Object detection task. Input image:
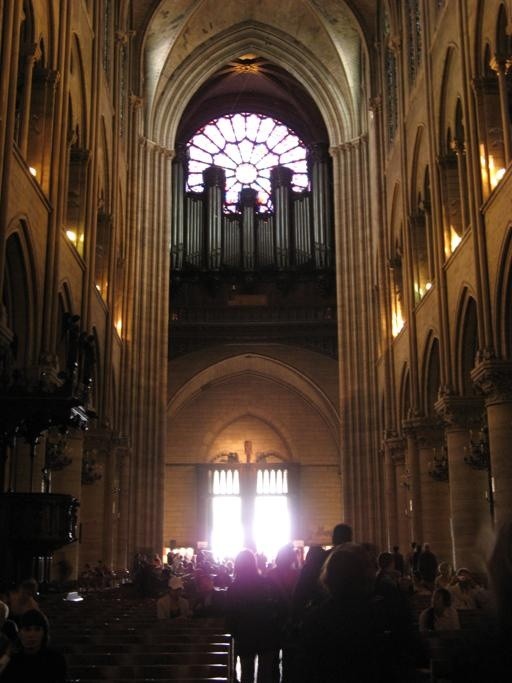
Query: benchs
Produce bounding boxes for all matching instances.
[0,583,239,683]
[406,590,481,683]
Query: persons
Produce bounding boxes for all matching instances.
[223,521,511,682]
[1,575,67,683]
[130,546,234,621]
[81,560,115,593]
